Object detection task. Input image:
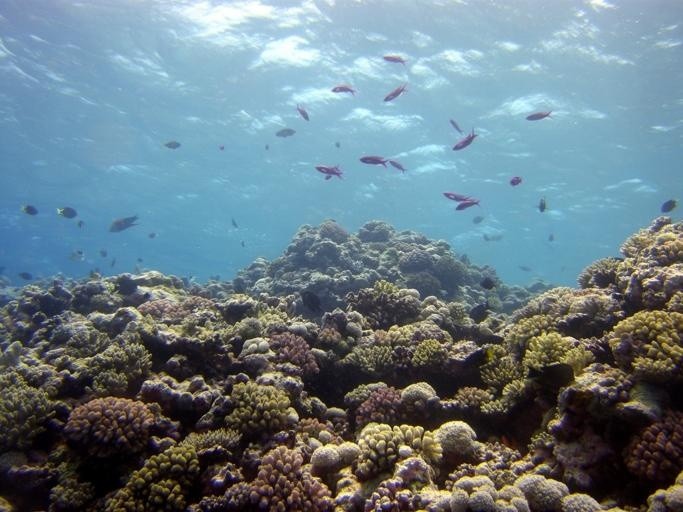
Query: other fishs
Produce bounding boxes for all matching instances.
[443,176,556,242]
